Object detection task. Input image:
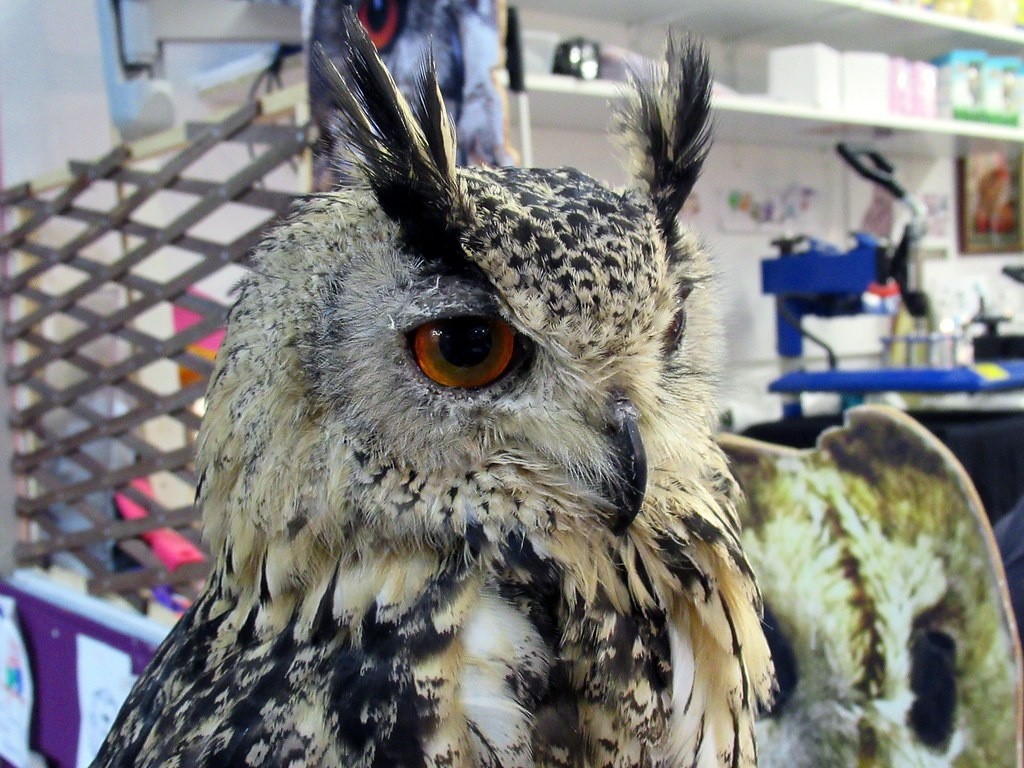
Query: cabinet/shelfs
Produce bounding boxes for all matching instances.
[509,1,1023,428]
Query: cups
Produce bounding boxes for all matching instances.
[553,38,600,74]
